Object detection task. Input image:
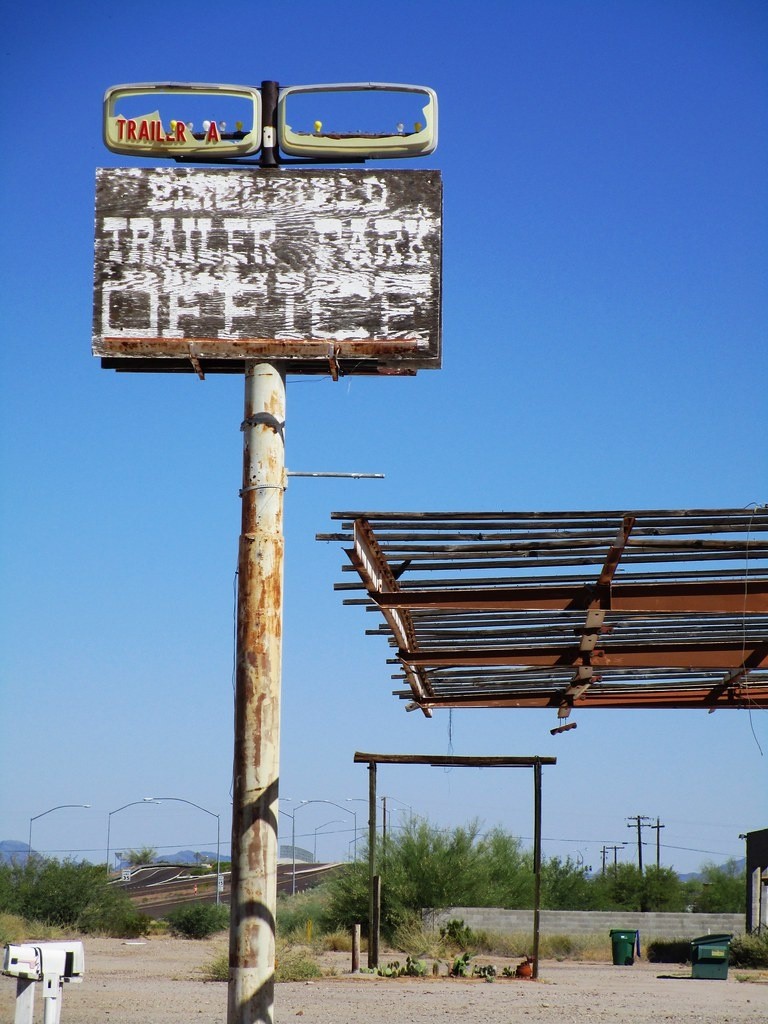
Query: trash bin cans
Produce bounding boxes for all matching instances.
[608,929,641,966]
[690,933,734,980]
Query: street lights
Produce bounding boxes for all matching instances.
[392,807,426,823]
[28,804,91,856]
[230,802,296,896]
[346,798,391,838]
[301,800,358,865]
[376,795,413,818]
[143,796,221,906]
[105,801,161,877]
